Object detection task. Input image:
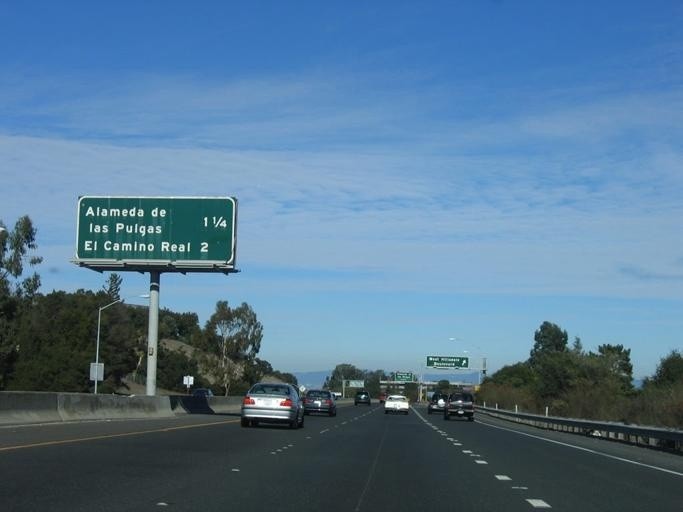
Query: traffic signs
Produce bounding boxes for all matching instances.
[427,356,469,369]
[75,196,235,266]
[395,372,413,382]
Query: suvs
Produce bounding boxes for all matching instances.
[428,393,448,414]
[444,393,475,421]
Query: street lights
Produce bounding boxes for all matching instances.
[95,295,149,393]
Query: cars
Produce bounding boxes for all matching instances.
[384,395,409,415]
[355,391,370,405]
[241,384,304,428]
[303,390,336,415]
[192,388,214,397]
